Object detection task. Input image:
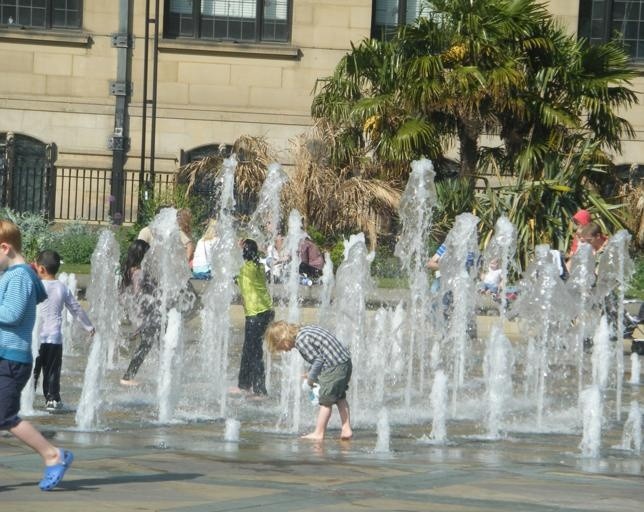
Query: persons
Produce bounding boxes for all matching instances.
[34,249,95,411]
[119,209,323,399]
[266,320,352,440]
[429,210,613,336]
[0,220,74,490]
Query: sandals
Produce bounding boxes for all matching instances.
[39,449,74,492]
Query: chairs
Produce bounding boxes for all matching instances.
[605,300,643,339]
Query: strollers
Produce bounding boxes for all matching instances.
[584,281,644,355]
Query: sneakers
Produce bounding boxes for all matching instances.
[45,400,63,411]
